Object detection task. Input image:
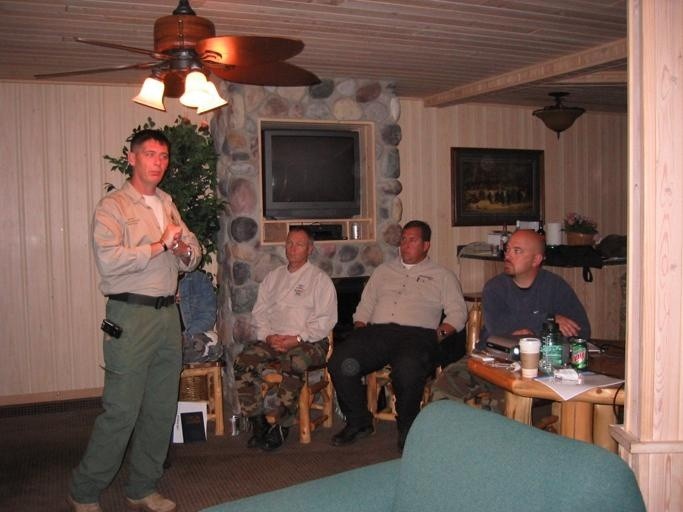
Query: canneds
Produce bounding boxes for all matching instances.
[568,337,589,373]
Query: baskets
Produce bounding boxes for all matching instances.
[179,367,219,426]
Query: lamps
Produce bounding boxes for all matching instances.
[131,67,166,111]
[180,61,210,107]
[196,80,228,115]
[532,92,586,140]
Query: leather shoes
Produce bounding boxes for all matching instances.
[122,491,176,512]
[64,491,104,512]
[330,417,376,447]
[397,432,410,452]
[246,414,270,448]
[263,423,288,452]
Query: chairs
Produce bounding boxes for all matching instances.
[466,305,489,409]
[261,329,334,444]
[365,364,442,421]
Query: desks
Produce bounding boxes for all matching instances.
[466,357,624,455]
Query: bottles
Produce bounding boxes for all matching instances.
[539,318,563,373]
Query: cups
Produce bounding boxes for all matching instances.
[518,338,540,378]
[544,223,563,246]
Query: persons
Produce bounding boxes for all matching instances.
[430,229,591,416]
[163,268,217,469]
[327,220,469,454]
[66,128,202,512]
[234,227,338,450]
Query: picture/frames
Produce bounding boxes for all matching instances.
[451,146,545,226]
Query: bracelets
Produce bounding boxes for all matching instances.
[184,246,192,257]
[160,240,168,251]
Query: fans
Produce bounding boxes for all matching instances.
[33,1,322,98]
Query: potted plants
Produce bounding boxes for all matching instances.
[104,115,235,401]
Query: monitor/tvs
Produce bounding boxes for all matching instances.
[261,129,361,220]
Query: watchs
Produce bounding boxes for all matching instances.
[438,328,448,339]
[297,335,303,345]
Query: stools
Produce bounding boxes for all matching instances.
[173,400,209,444]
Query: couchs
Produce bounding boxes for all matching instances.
[198,399,646,512]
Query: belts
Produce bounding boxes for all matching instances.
[106,290,176,310]
